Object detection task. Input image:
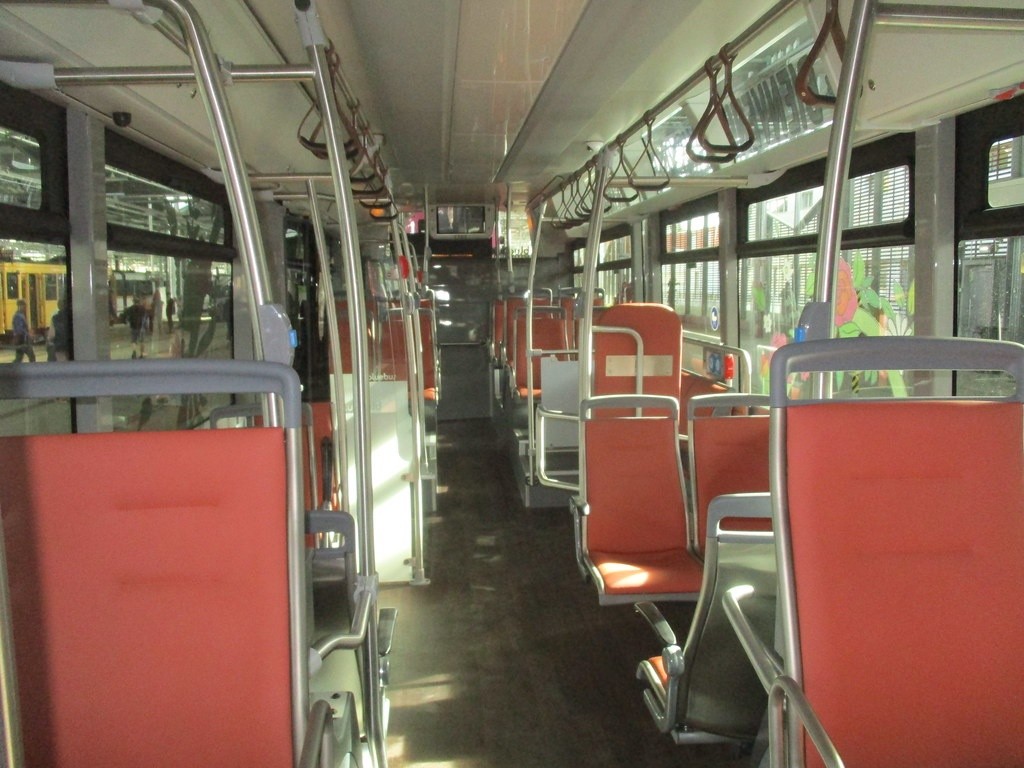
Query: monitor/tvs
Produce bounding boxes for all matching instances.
[428,203,495,240]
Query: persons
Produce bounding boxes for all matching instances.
[12,300,35,363]
[110,290,176,360]
[48,298,70,361]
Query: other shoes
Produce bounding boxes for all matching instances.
[132,351,136,359]
[139,355,144,359]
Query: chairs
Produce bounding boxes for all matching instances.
[489,287,1024,768]
[0,239,440,768]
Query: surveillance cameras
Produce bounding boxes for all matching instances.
[111,112,132,127]
[587,142,603,152]
